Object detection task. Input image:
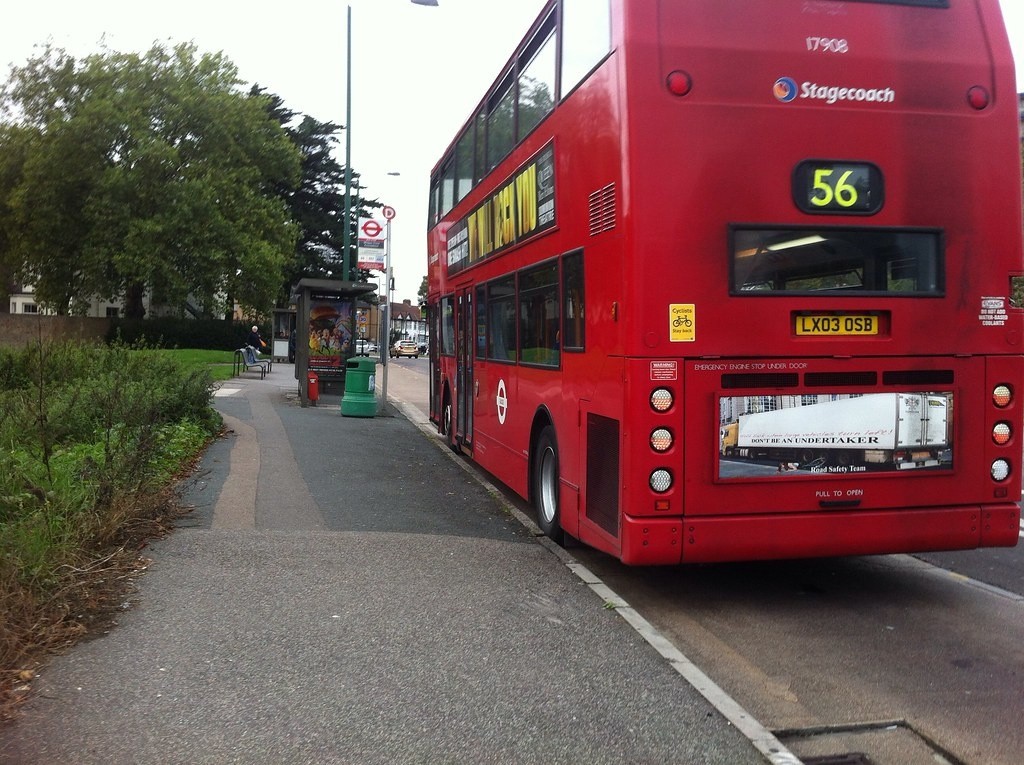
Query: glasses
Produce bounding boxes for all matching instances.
[344,342,349,345]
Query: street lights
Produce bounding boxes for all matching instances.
[343,0,440,282]
[355,172,401,283]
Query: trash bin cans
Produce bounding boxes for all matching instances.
[340,356,378,417]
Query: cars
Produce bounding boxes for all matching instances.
[356,339,369,357]
[369,343,380,352]
[389,340,419,359]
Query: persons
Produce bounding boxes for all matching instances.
[247,326,262,363]
[310,327,342,356]
[285,331,297,364]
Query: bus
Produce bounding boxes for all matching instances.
[420,0,1024,568]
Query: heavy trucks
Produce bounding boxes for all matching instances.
[722,391,948,471]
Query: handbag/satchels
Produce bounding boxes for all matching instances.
[260,339,266,347]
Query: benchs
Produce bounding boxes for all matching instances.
[233,348,266,380]
[246,345,272,373]
